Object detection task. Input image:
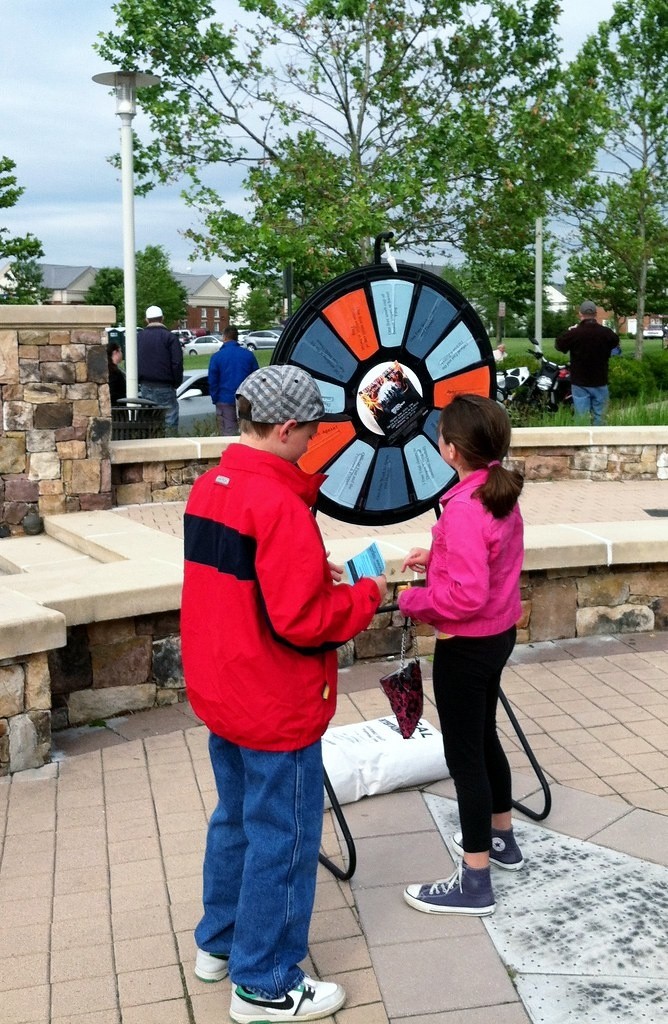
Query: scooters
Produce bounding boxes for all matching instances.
[497,366,531,409]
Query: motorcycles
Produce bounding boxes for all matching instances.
[523,334,576,412]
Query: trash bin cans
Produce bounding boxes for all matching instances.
[111,398,169,440]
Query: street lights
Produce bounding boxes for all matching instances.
[91,66,167,421]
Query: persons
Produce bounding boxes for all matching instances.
[208,325,259,436]
[107,340,126,406]
[137,306,183,438]
[359,372,408,415]
[555,301,621,424]
[493,344,507,362]
[178,364,387,1024]
[397,392,526,917]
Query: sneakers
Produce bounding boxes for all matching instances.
[403,859,496,917]
[452,826,524,871]
[229,969,347,1024]
[194,949,231,983]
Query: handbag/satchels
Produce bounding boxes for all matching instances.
[380,615,424,739]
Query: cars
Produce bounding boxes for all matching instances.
[183,334,248,356]
[170,327,250,346]
[172,369,238,418]
[642,324,665,339]
[242,329,284,352]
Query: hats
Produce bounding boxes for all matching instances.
[146,305,162,319]
[234,365,353,424]
[580,301,596,314]
